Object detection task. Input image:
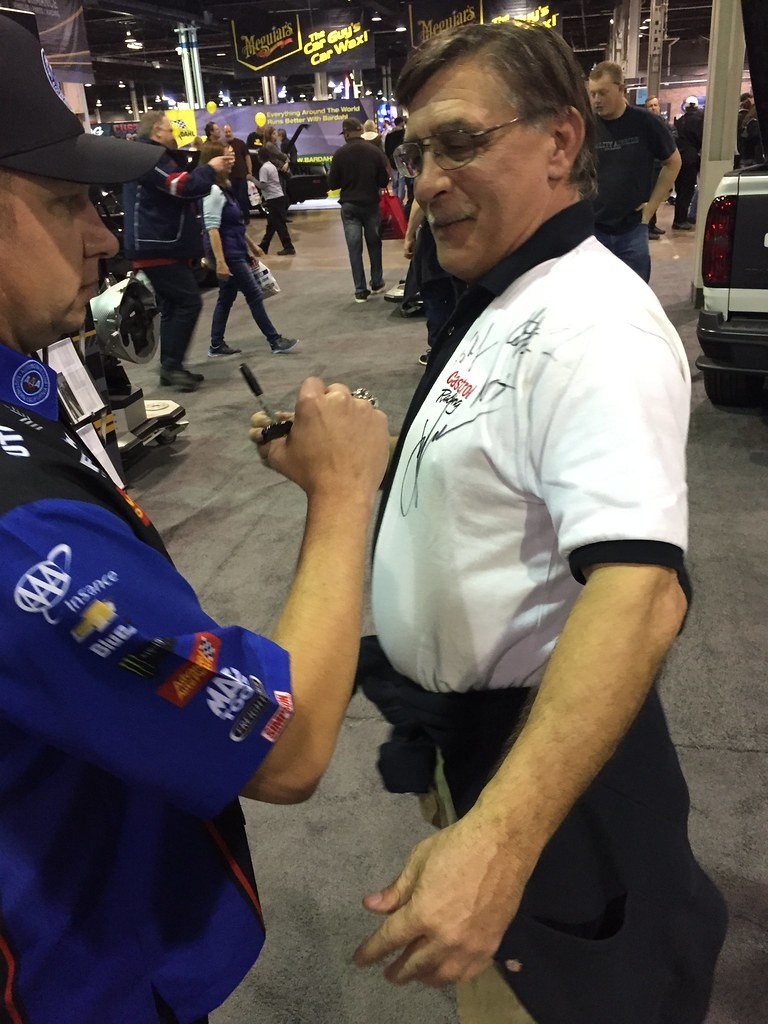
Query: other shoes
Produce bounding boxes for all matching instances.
[672,221,692,231]
[159,365,204,389]
[648,225,665,240]
[276,248,296,256]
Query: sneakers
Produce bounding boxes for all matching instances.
[419,348,432,365]
[270,335,301,354]
[355,289,371,304]
[208,341,242,359]
[371,281,386,294]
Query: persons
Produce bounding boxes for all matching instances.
[587,61,682,283]
[359,115,414,210]
[190,121,311,358]
[249,22,730,1024]
[403,197,468,365]
[124,111,237,392]
[643,92,764,241]
[1,6,388,1024]
[327,118,391,303]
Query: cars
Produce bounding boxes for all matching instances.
[247,121,332,218]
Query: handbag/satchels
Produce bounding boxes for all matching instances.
[376,186,408,241]
[248,255,282,301]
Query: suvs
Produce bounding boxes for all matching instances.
[690,166,767,411]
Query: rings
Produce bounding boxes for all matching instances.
[350,388,379,409]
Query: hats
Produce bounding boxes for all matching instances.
[1,11,169,185]
[684,95,698,108]
[338,117,363,137]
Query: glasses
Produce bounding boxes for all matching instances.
[389,107,560,179]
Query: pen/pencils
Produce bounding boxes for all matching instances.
[260,419,292,441]
[238,362,274,420]
